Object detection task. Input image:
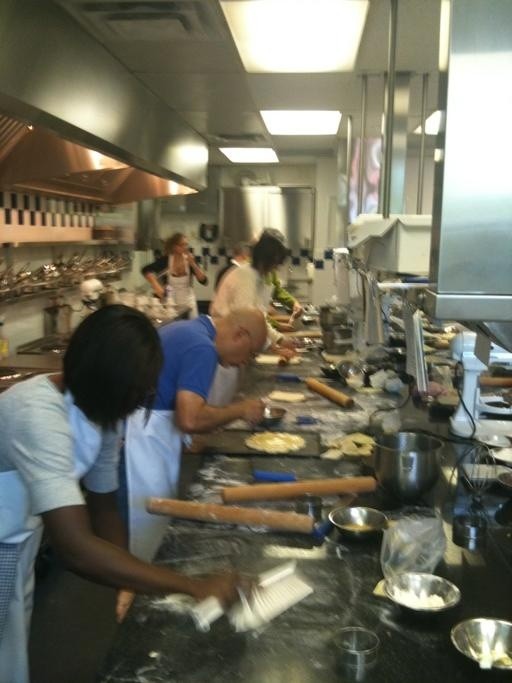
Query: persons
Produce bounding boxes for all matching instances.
[201,226,302,469]
[142,232,209,320]
[80,304,268,637]
[0,303,259,683]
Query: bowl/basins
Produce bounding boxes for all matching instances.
[337,358,365,380]
[256,405,286,426]
[318,363,338,379]
[383,571,464,616]
[323,326,353,353]
[446,616,512,675]
[320,306,346,330]
[327,503,386,539]
[374,431,446,498]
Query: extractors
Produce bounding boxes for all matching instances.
[2,2,209,206]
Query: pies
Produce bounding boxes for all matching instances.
[245,431,307,453]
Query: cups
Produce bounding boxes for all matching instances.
[329,626,381,682]
[295,493,322,521]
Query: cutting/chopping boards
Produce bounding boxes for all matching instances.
[278,330,322,339]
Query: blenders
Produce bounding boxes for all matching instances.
[449,328,511,446]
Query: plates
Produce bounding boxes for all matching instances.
[383,312,461,411]
[453,536,489,550]
[463,390,512,486]
[453,515,488,537]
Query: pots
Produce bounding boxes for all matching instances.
[2,248,139,298]
